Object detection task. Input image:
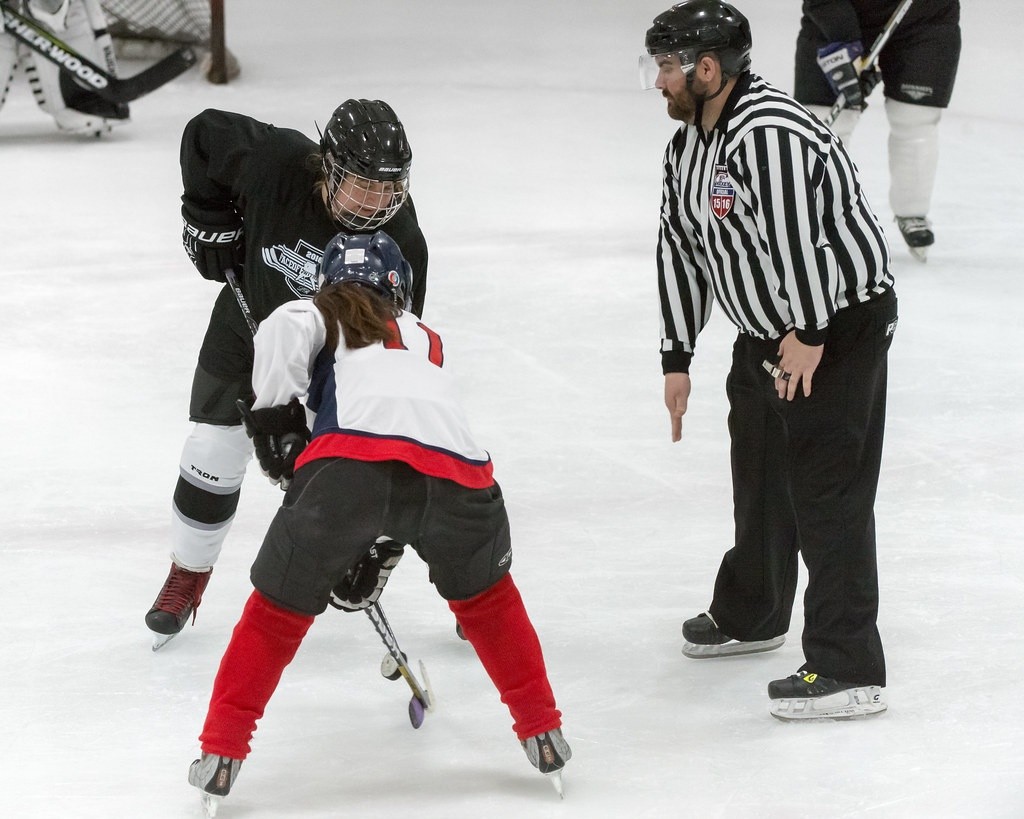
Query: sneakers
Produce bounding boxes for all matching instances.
[145,561,213,652]
[521,726,572,799]
[893,215,935,263]
[682,611,787,659]
[188,751,243,819]
[768,664,887,718]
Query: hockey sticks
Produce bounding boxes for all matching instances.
[0,1,199,107]
[58,0,131,119]
[823,1,914,128]
[225,264,425,732]
[235,398,441,714]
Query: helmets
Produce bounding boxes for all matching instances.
[319,230,413,309]
[645,0,752,78]
[314,99,416,230]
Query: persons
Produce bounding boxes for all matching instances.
[187,229,573,817]
[145,98,428,652]
[638,0,899,721]
[793,0,961,261]
[0,1,122,138]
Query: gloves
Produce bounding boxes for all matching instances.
[181,194,246,283]
[816,40,882,104]
[329,539,404,612]
[236,397,312,491]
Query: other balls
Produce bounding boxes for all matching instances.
[381,650,408,681]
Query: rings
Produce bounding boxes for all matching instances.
[762,359,792,381]
